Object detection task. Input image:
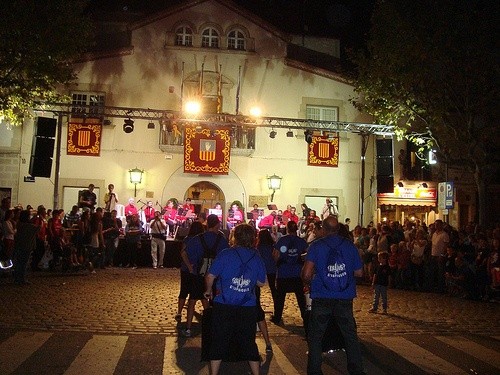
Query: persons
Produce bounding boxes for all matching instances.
[0,198,500,375]
[80,183,96,211]
[104,184,118,212]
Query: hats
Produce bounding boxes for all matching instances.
[207,214,222,224]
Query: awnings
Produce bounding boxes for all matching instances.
[377,197,436,207]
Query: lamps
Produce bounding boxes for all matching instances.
[327,130,334,140]
[422,182,428,189]
[286,128,293,137]
[303,129,313,145]
[147,119,155,130]
[397,181,404,188]
[128,165,145,197]
[122,117,134,134]
[266,172,283,202]
[164,154,173,160]
[268,127,277,138]
[210,125,218,137]
[195,122,202,129]
[227,125,235,136]
[103,117,112,126]
[167,121,174,134]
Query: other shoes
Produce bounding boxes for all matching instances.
[183,328,191,336]
[89,271,96,274]
[176,315,181,320]
[368,308,377,313]
[266,346,272,352]
[88,262,93,269]
[153,266,157,268]
[270,315,281,322]
[160,265,163,268]
[381,308,386,313]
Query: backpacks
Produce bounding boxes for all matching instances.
[199,233,222,281]
[318,237,351,292]
[221,246,258,305]
[282,237,302,264]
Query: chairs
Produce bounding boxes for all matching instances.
[138,210,170,238]
[115,204,127,226]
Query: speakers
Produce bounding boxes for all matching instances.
[375,138,394,194]
[28,116,57,178]
